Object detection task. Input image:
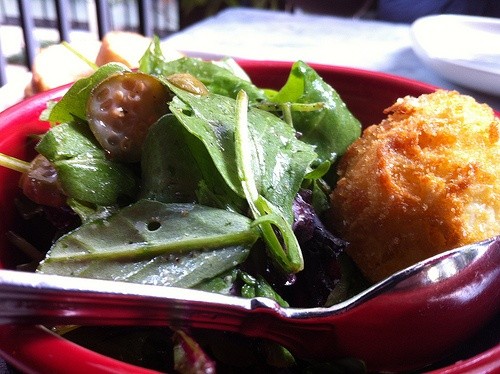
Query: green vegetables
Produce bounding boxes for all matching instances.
[0,30,364,374]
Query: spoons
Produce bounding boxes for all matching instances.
[0,234,499,373]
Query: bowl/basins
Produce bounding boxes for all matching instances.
[1,57,500,373]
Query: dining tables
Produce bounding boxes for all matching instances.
[0,8,500,373]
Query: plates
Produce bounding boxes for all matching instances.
[411,13,500,96]
